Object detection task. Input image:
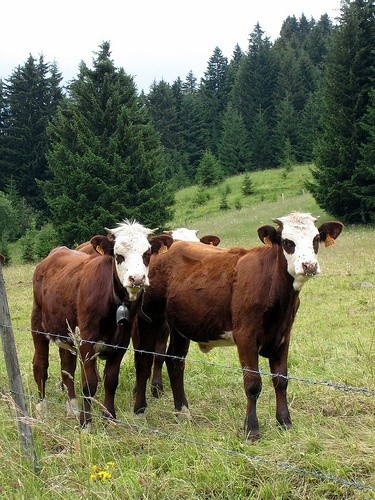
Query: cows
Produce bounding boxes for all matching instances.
[30,211,344,445]
